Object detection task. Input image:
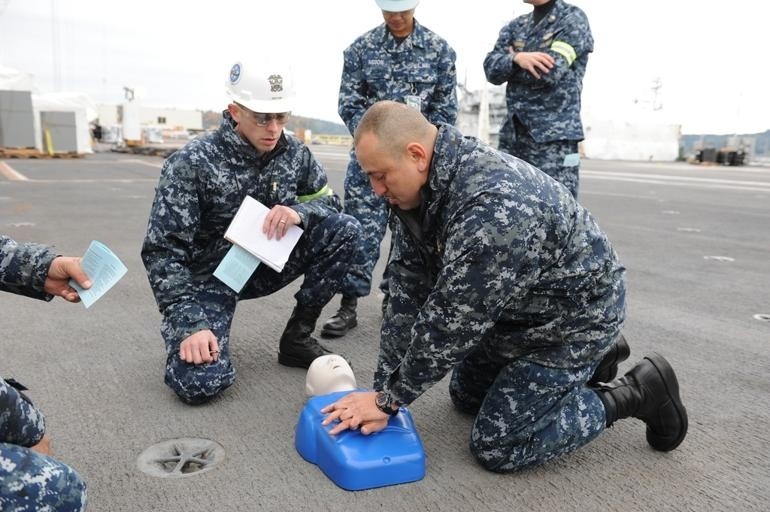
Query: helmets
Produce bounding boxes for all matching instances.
[374,0,420,13]
[226,58,300,115]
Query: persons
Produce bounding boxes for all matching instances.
[319,101,688,476]
[0,230,92,512]
[295,353,426,490]
[138,59,363,407]
[321,0,460,339]
[481,0,595,201]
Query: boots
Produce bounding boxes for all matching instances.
[320,295,360,339]
[593,334,631,384]
[590,352,690,452]
[277,305,355,377]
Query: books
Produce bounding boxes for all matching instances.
[68,238,128,309]
[212,243,262,294]
[222,193,305,274]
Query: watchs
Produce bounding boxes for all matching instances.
[374,390,399,417]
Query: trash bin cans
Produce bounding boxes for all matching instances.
[696,147,746,166]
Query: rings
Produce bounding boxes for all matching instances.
[280,218,287,224]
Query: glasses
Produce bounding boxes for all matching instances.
[230,102,292,127]
